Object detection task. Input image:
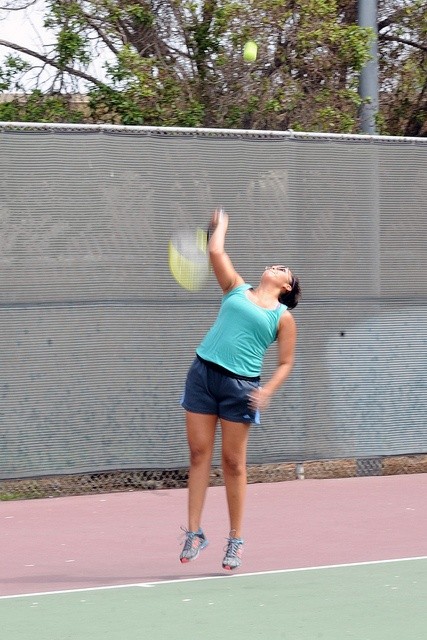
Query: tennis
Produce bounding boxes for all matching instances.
[243,42,257,62]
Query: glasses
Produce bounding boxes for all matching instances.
[265,266,290,284]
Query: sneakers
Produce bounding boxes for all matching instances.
[222,530,243,569]
[179,526,208,563]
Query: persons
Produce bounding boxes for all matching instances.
[180,208,299,570]
